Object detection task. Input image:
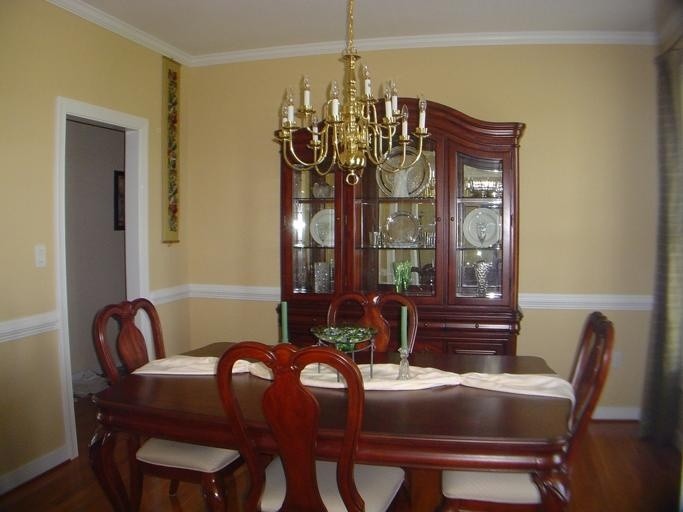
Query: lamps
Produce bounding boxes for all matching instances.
[274,1,433,187]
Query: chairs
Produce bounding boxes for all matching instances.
[90,286,615,511]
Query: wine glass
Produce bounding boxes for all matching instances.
[401,259,412,289]
[392,261,404,290]
[476,223,487,248]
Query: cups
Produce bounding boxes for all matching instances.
[368,231,390,248]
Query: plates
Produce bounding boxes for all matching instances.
[376,145,432,197]
[309,208,335,247]
[462,207,502,248]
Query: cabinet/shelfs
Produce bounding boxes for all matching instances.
[276,96,527,356]
